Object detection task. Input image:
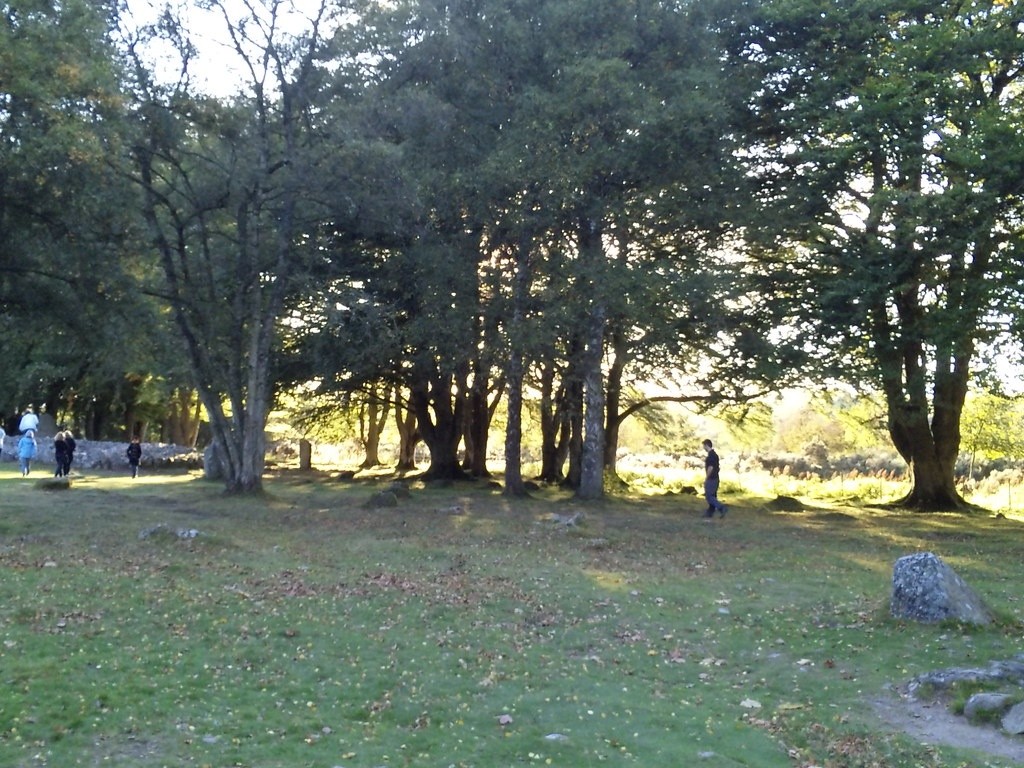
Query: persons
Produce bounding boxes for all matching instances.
[701,439,727,518]
[54,431,69,477]
[19,411,39,435]
[127,436,142,479]
[64,430,75,476]
[17,430,38,476]
